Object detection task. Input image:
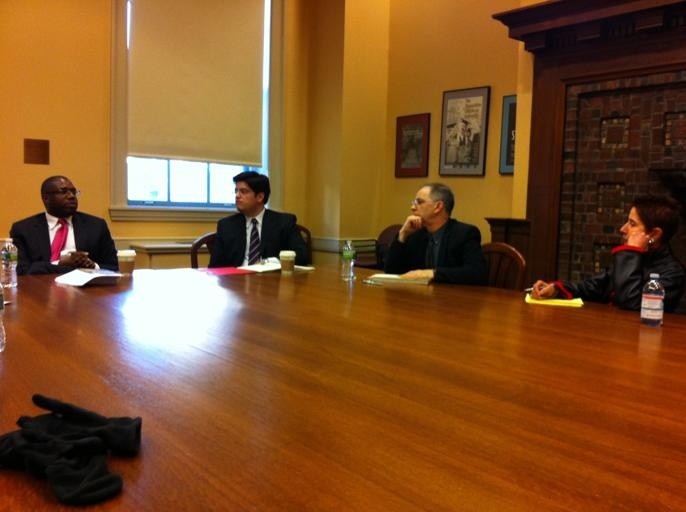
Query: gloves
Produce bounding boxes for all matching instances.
[0,416,123,507]
[18,393,141,459]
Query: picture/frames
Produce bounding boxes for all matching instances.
[394,112,431,178]
[498,94,517,175]
[438,85,491,177]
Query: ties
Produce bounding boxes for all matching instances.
[248,217,260,266]
[50,219,69,262]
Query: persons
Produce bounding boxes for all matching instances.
[10,176,119,274]
[459,119,467,163]
[209,171,312,267]
[385,182,488,285]
[465,128,474,149]
[446,123,458,163]
[532,198,679,313]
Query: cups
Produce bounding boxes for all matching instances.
[117,249,137,274]
[279,250,297,276]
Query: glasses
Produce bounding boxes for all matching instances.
[43,189,81,198]
[410,199,435,206]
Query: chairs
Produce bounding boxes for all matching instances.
[189,231,217,268]
[481,240,527,289]
[295,223,313,266]
[354,222,403,269]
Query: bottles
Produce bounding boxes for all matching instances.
[341,240,357,281]
[0,284,7,353]
[640,273,665,327]
[0,238,18,289]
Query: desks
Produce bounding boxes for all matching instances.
[129,240,209,270]
[0,263,686,511]
